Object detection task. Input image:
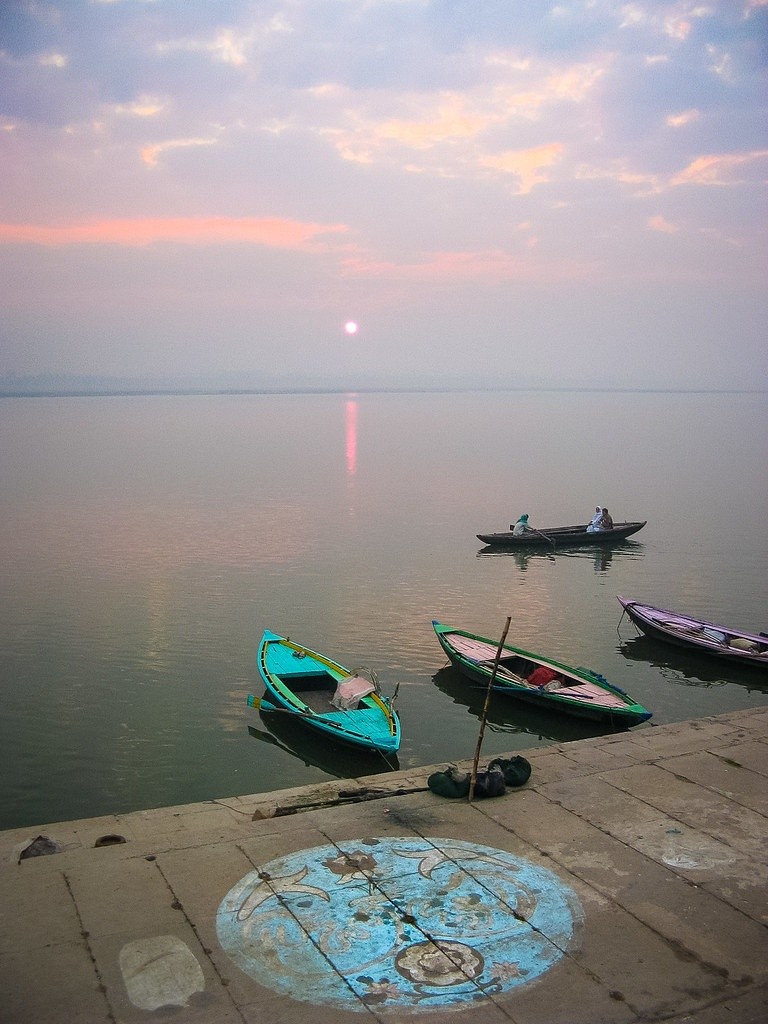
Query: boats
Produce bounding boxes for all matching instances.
[432,620,653,719]
[476,521,647,544]
[616,595,768,670]
[257,630,401,753]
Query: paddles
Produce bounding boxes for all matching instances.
[473,685,594,700]
[707,649,767,658]
[245,694,343,726]
[529,526,554,542]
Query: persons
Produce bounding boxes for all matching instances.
[586,505,614,532]
[512,514,536,536]
